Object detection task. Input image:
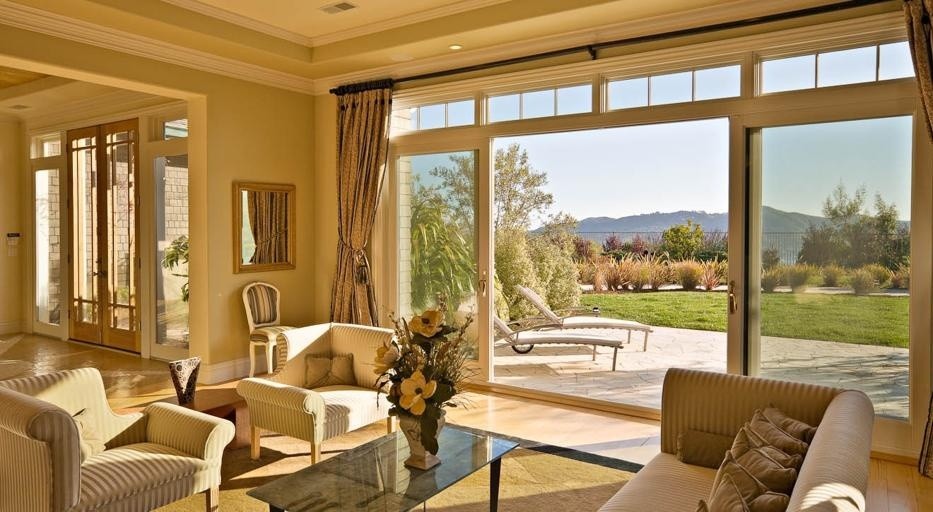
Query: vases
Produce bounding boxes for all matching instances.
[399,410,448,470]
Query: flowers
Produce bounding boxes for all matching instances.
[371,294,477,458]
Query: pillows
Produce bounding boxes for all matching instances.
[673,397,820,512]
[303,353,354,389]
[70,407,105,464]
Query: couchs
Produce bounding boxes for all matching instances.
[596,365,876,511]
[229,321,397,465]
[1,365,236,511]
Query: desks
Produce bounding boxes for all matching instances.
[111,387,249,429]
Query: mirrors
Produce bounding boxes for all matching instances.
[230,180,296,272]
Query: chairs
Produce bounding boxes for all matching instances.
[493,313,625,370]
[513,284,655,350]
[242,281,295,377]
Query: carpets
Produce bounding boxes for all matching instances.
[147,410,635,512]
